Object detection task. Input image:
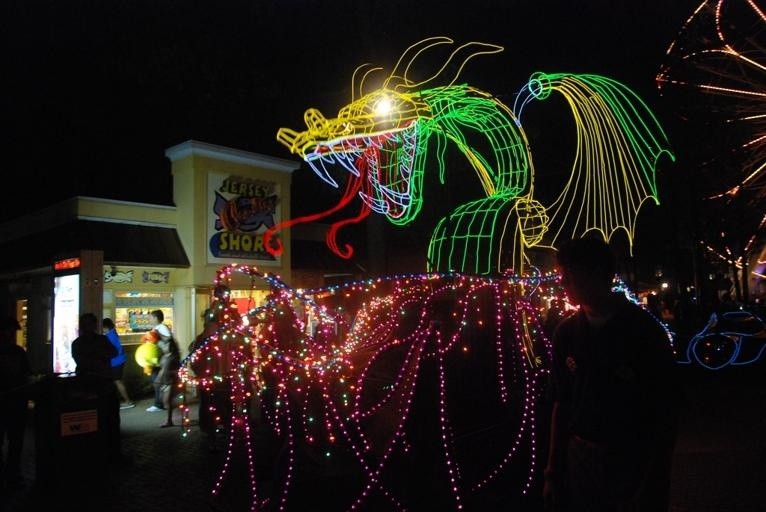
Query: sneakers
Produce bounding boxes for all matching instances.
[119,403,135,409]
[159,419,173,427]
[145,406,163,412]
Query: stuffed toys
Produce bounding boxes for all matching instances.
[134,330,160,376]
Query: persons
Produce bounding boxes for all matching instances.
[181,305,355,444]
[0,311,33,489]
[153,341,190,429]
[639,276,766,334]
[546,236,692,512]
[102,317,136,410]
[144,309,177,412]
[71,312,134,466]
